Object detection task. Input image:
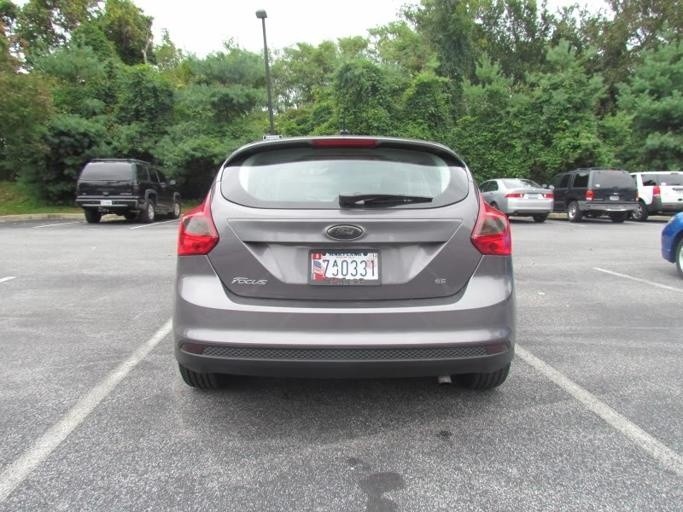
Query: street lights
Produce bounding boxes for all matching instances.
[255,10,277,136]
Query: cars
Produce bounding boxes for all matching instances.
[661,212,683,273]
[173,135,514,388]
[630,171,683,220]
[477,177,555,222]
[75,159,181,224]
[545,166,639,222]
